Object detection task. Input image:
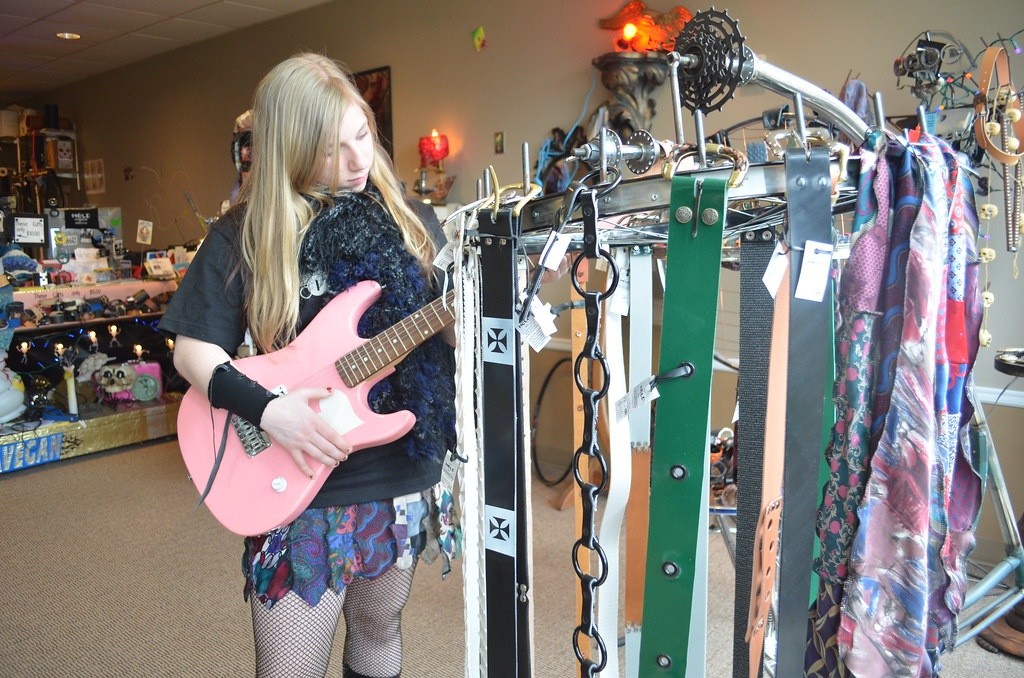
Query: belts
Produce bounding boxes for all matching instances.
[974,47,1024,166]
[438,113,831,678]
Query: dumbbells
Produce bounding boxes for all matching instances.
[570,128,665,175]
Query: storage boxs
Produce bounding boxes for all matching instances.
[93,361,163,402]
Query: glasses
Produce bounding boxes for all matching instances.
[711,454,732,477]
[711,444,734,461]
[711,481,738,508]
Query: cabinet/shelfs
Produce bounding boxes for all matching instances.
[0,279,189,472]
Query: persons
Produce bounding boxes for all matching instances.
[156,52,569,678]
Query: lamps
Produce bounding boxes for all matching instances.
[592,0,694,139]
[413,129,456,206]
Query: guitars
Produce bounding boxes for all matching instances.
[175,250,578,539]
